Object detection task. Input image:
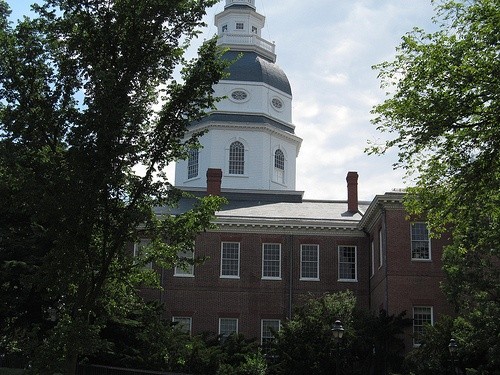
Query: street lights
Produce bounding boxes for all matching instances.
[449,339,457,375]
[332,320,345,375]
